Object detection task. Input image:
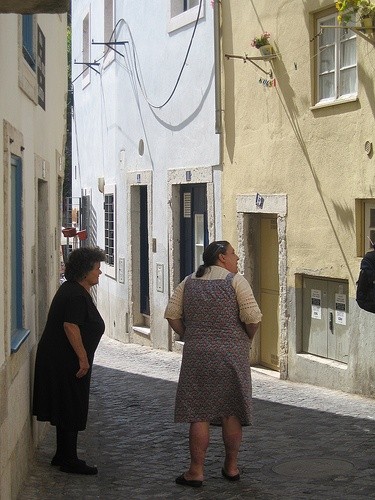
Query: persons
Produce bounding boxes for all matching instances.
[165,241,263,487]
[356,251,375,314]
[32,247,105,475]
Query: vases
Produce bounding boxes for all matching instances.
[258,45,273,55]
[360,18,373,26]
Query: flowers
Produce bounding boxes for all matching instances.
[335,0,375,25]
[250,32,271,49]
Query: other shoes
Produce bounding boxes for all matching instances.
[61,459,98,474]
[51,455,86,466]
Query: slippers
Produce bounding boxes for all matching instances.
[176,474,203,487]
[221,467,239,480]
